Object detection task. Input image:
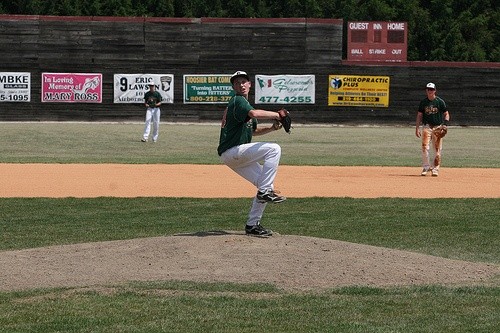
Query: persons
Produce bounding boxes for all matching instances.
[217,71,292,237]
[416,83,450,176]
[141,83,163,143]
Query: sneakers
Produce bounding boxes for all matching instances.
[256,188,286,204]
[245,220,273,238]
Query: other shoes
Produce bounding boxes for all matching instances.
[152,138,156,143]
[141,139,148,142]
[421,167,438,176]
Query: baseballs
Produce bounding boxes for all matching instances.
[273,122,281,130]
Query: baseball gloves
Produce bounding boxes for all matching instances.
[277,109,292,135]
[432,124,448,139]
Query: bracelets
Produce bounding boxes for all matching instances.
[442,120,449,126]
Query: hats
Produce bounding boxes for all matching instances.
[230,71,248,82]
[426,83,436,89]
[148,82,154,85]
